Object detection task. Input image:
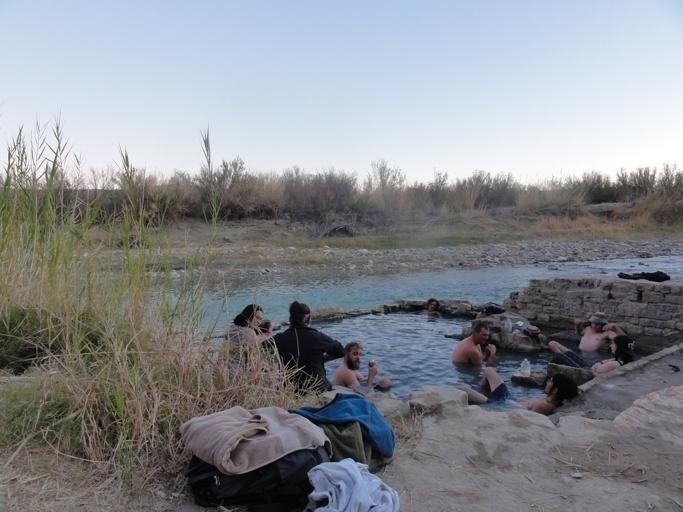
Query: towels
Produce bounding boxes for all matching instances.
[177,404,333,476]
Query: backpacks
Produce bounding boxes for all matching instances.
[186,443,331,508]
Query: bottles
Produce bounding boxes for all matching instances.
[520,358,531,378]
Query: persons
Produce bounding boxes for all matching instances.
[332,343,391,395]
[456,366,576,414]
[262,301,345,393]
[452,321,496,369]
[422,297,442,317]
[574,315,627,356]
[255,319,273,343]
[222,302,263,371]
[549,335,634,376]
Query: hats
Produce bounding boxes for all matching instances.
[554,373,577,398]
[613,335,635,358]
[589,311,608,324]
